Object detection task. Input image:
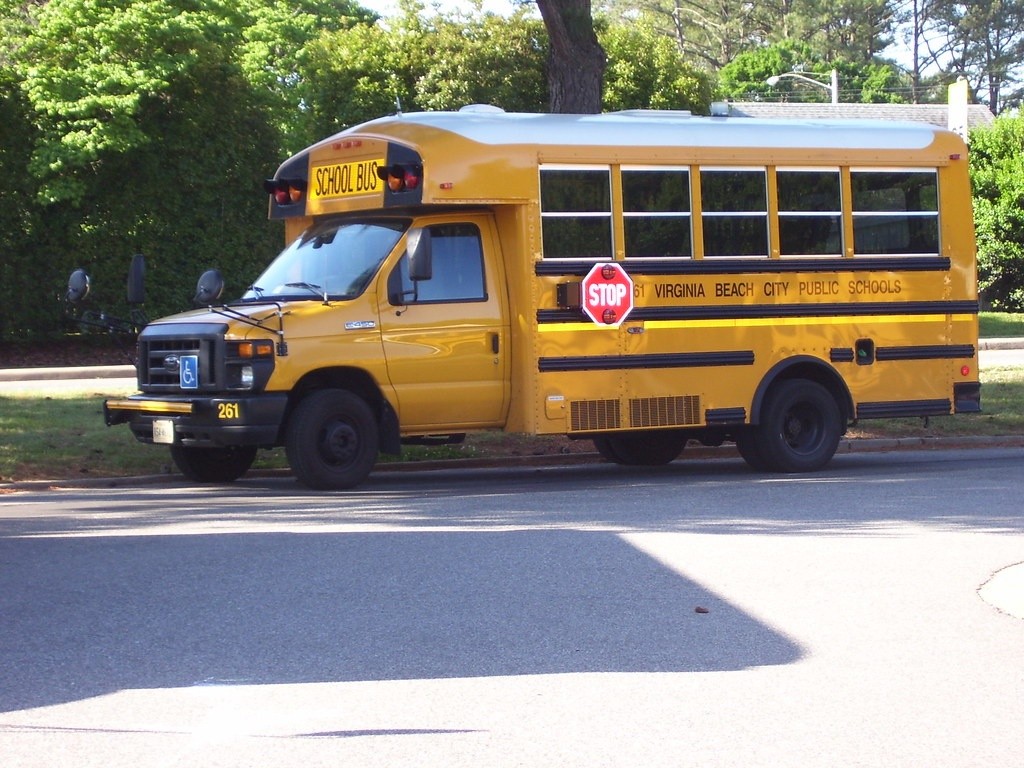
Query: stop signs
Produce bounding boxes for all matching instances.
[582,262,635,328]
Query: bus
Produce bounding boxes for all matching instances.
[63,104,982,492]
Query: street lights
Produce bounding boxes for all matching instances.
[767,67,840,104]
